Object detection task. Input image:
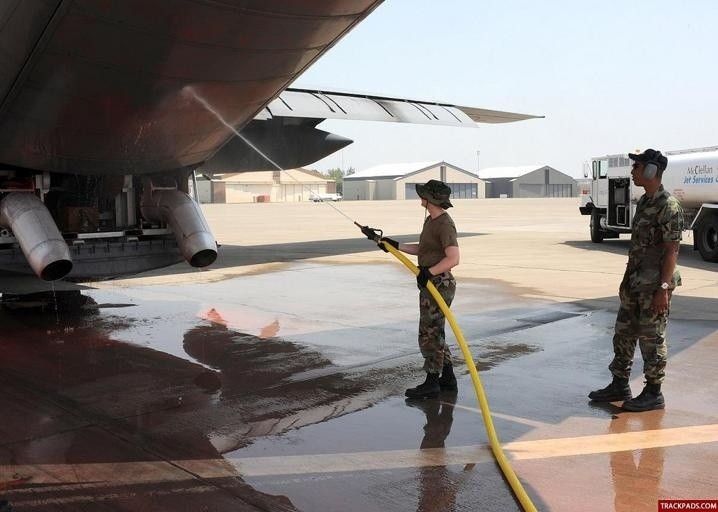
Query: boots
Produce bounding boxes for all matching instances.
[587,375,665,412]
[405,365,457,398]
[405,388,458,414]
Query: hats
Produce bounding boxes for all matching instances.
[415,179,453,210]
[628,149,668,170]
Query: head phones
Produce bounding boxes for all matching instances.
[643,151,660,181]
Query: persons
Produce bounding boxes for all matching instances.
[587,150,683,413]
[378,179,460,400]
[403,396,458,512]
[588,398,665,512]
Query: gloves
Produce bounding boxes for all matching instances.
[377,237,399,253]
[416,266,433,287]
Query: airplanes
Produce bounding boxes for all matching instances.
[0,0,546,282]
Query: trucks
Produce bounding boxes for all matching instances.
[579,146,717,262]
[308,193,342,202]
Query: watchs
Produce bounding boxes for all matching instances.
[655,282,673,290]
